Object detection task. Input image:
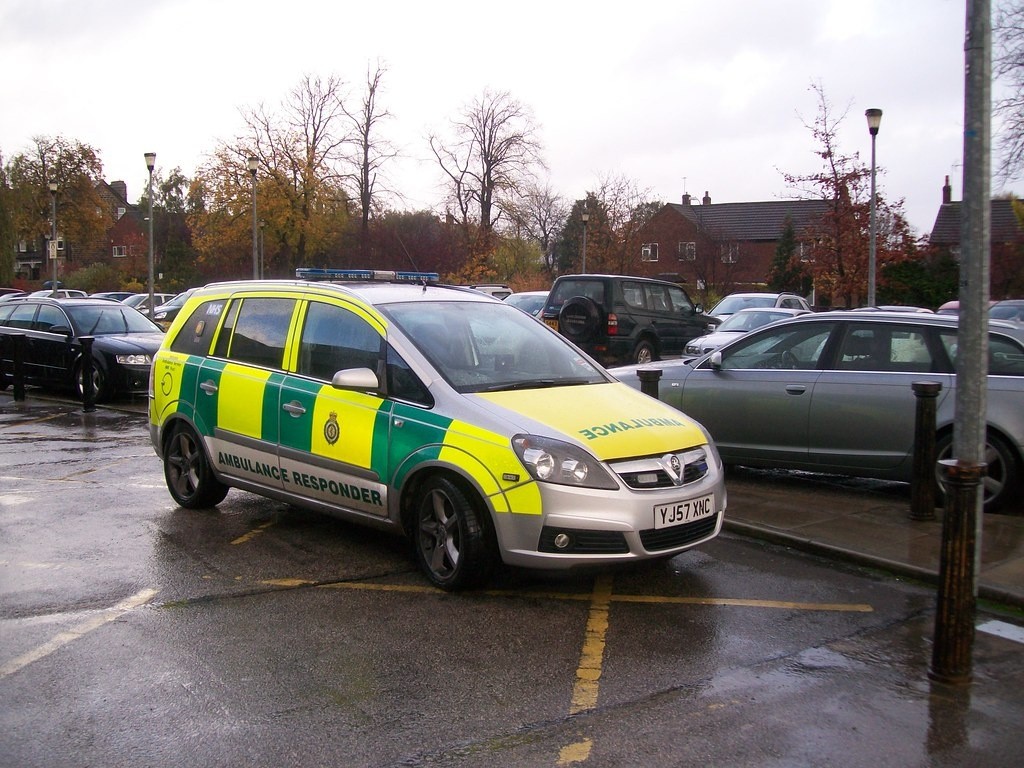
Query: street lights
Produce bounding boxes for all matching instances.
[580,205,590,274]
[49,177,57,299]
[145,152,156,324]
[865,108,883,305]
[248,155,259,278]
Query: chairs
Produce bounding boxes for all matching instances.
[416,323,452,368]
[624,290,636,305]
[594,289,604,301]
[752,314,771,327]
[844,336,893,371]
[648,295,666,310]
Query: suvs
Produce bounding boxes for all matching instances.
[535,272,729,367]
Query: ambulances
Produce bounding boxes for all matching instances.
[146,264,727,593]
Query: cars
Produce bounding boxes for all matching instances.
[0,297,174,402]
[689,283,1024,382]
[0,288,563,347]
[605,310,1024,509]
